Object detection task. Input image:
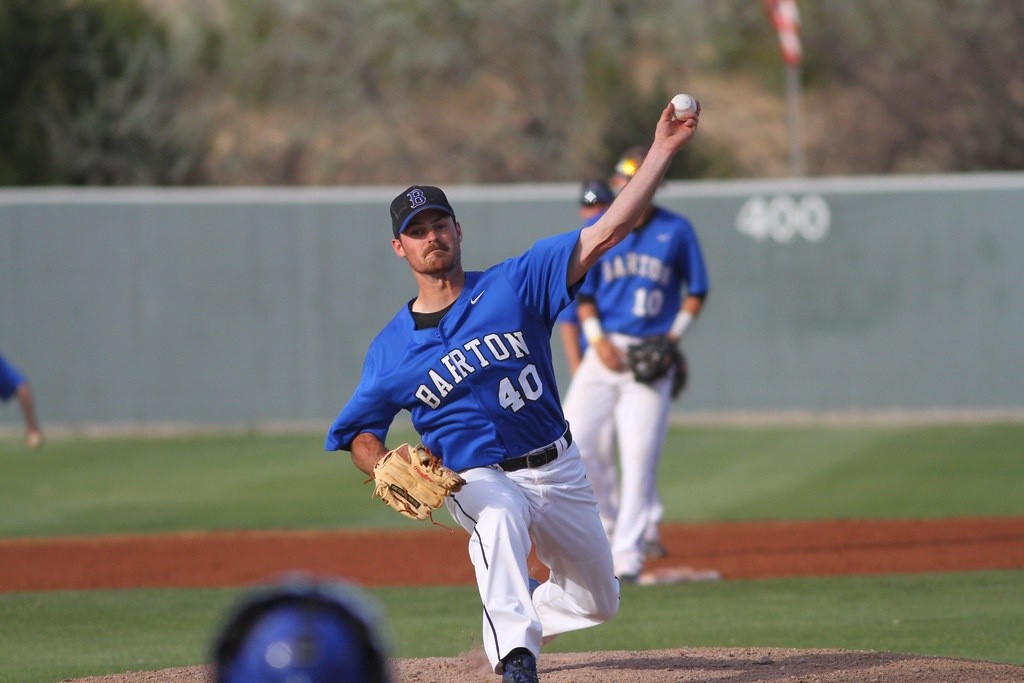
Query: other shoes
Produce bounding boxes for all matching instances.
[642,539,668,559]
[617,574,637,585]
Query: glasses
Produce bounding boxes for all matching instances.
[615,158,640,177]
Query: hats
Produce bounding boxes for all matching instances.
[579,180,614,205]
[614,145,649,181]
[389,186,457,238]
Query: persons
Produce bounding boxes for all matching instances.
[326,98,701,683]
[561,148,708,581]
[0,355,42,447]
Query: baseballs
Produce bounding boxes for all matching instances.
[670,94,698,122]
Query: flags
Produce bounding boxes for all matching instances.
[765,0,803,64]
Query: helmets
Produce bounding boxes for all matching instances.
[209,580,390,683]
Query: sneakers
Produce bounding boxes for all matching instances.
[528,577,540,599]
[502,649,539,683]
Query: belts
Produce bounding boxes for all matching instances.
[498,420,572,471]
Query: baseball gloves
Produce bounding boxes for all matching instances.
[628,337,677,382]
[372,443,468,522]
[672,349,686,401]
